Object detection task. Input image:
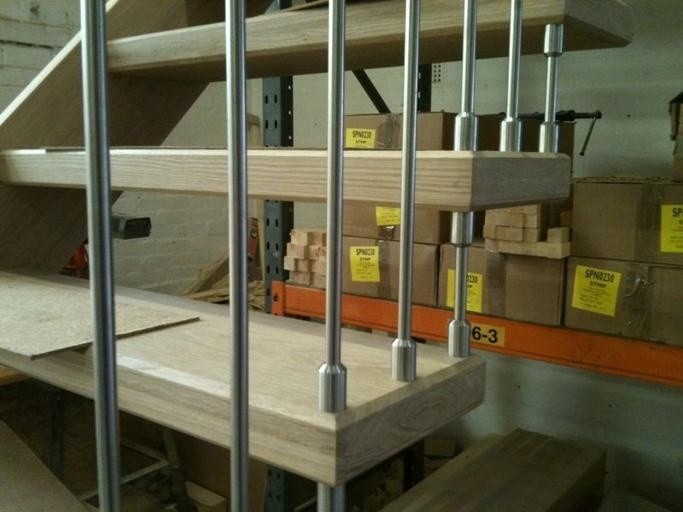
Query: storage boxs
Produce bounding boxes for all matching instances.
[341,93,681,345]
